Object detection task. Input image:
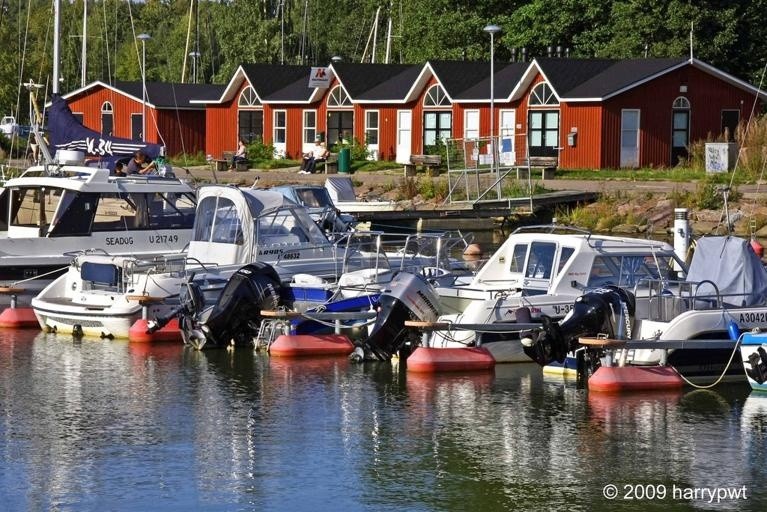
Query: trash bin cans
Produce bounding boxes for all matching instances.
[340,148,350,175]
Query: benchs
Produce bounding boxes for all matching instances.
[214,150,249,171]
[399,155,442,178]
[517,156,560,180]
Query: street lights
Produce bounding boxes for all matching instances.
[483,24,503,175]
[134,30,156,144]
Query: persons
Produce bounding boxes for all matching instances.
[29,132,49,162]
[113,163,126,177]
[228,141,246,171]
[127,152,155,229]
[299,139,327,173]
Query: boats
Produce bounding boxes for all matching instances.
[737,328,767,396]
[348,203,696,364]
[139,221,497,352]
[30,183,470,339]
[0,161,341,319]
[513,185,767,383]
